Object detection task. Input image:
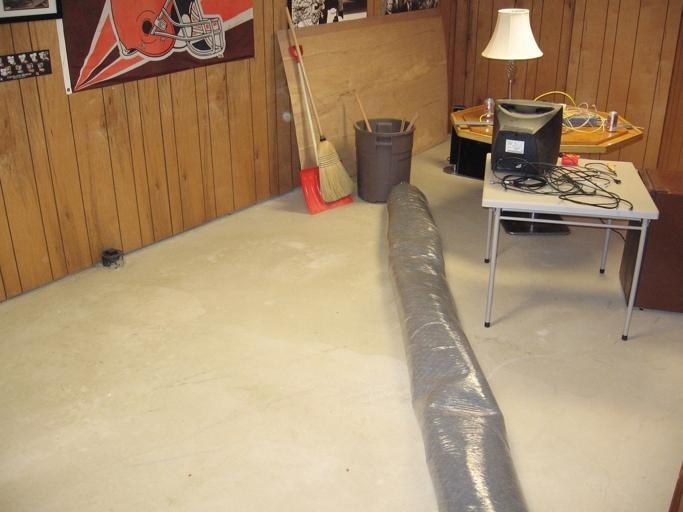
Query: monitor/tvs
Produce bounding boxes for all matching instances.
[490,99,564,175]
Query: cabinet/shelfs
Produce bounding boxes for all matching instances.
[617,166,683,313]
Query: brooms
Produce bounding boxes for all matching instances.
[283,5,356,203]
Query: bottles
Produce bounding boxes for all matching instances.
[606,110,619,132]
[484,97,495,118]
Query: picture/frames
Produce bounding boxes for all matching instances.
[0,0,64,23]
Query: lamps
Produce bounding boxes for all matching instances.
[481,8,543,100]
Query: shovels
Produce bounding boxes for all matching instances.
[289,44,353,215]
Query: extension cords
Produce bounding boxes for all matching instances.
[568,114,600,127]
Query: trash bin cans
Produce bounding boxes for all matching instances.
[354,119,416,205]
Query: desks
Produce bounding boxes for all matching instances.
[451,101,644,235]
[481,153,659,341]
